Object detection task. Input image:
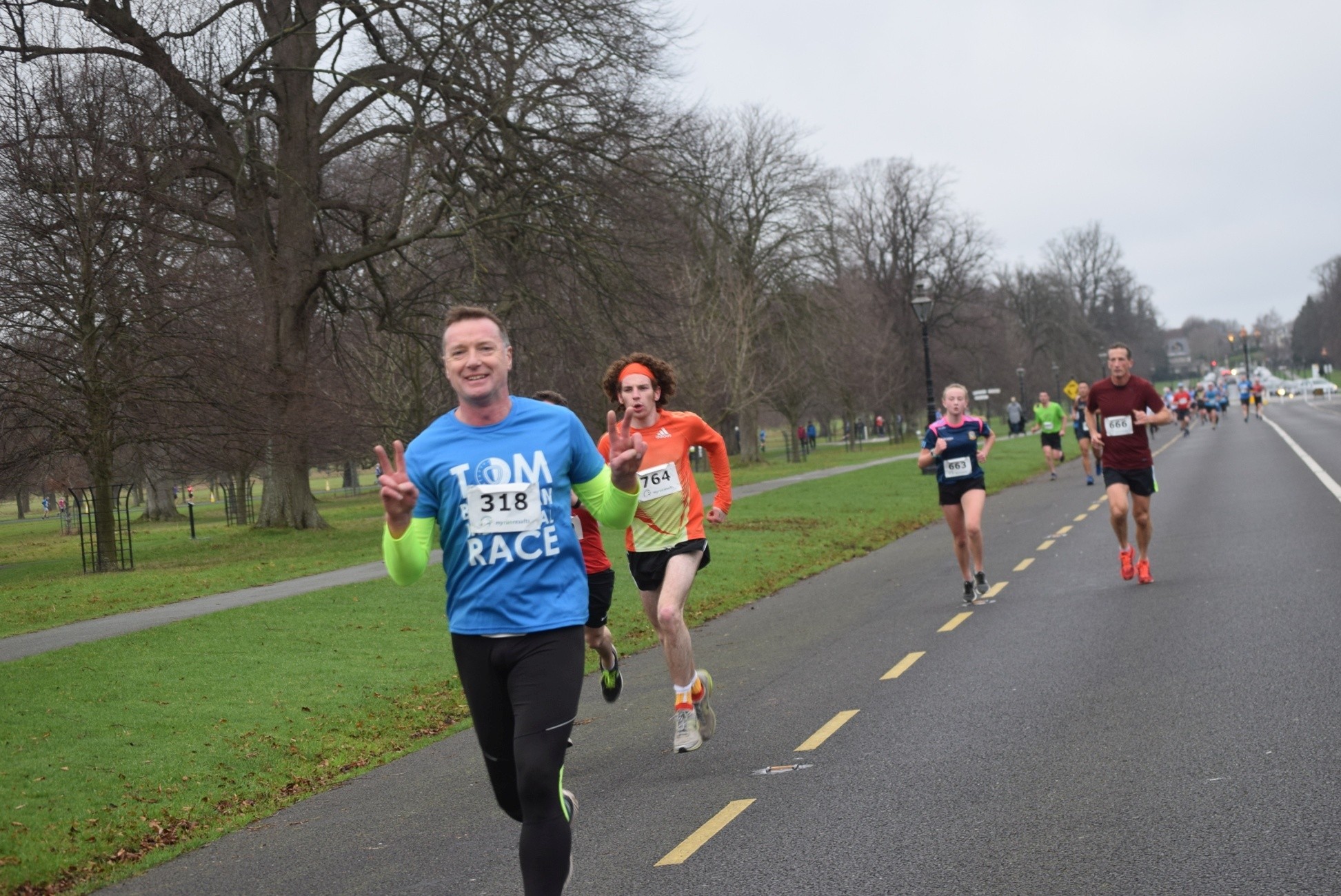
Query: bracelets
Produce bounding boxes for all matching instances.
[571,497,582,509]
[930,448,939,458]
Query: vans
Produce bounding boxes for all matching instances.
[1297,377,1337,394]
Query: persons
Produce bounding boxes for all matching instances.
[528,352,731,753]
[375,462,382,480]
[173,484,195,506]
[41,495,65,520]
[374,304,649,896]
[694,339,1265,604]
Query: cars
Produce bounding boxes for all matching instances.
[1221,368,1245,384]
[1204,372,1218,383]
[1270,382,1299,397]
[1254,367,1281,385]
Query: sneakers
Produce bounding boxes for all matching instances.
[673,703,702,754]
[1136,558,1155,584]
[562,788,579,891]
[962,575,977,602]
[1050,473,1057,480]
[1119,543,1138,580]
[1096,463,1101,475]
[599,644,624,704]
[692,669,715,741]
[973,571,991,595]
[1087,475,1094,485]
[1060,452,1065,463]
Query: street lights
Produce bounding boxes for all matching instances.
[1017,362,1026,412]
[1051,361,1061,406]
[909,282,938,475]
[1228,325,1260,379]
[1098,345,1108,379]
